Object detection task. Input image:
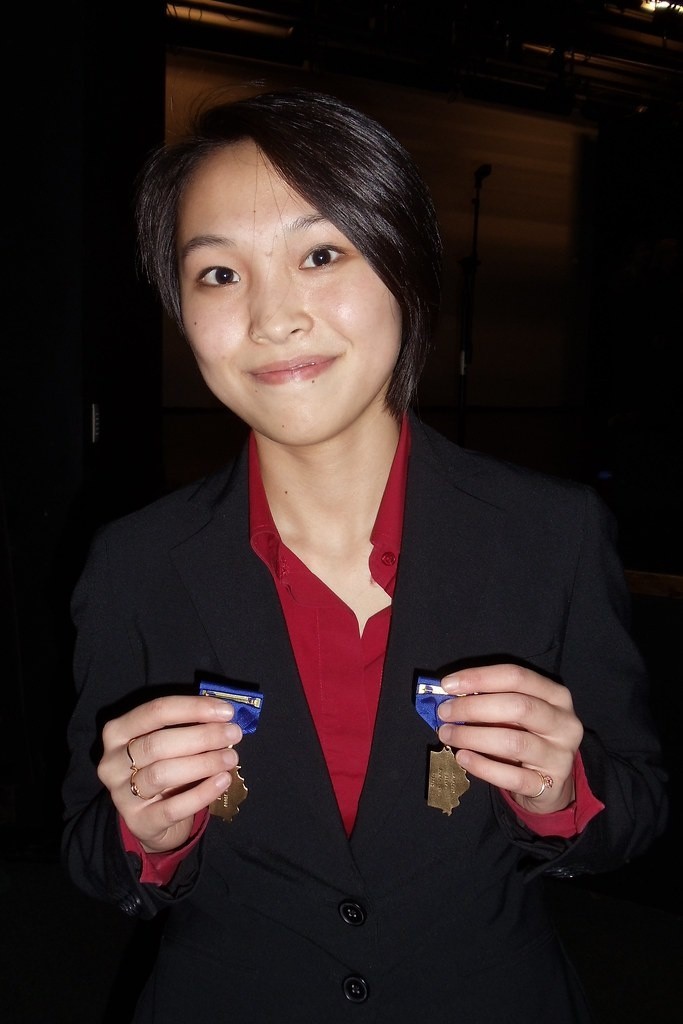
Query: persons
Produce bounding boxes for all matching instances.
[57,90,672,1024]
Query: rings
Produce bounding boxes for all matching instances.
[126,738,156,800]
[525,770,554,798]
